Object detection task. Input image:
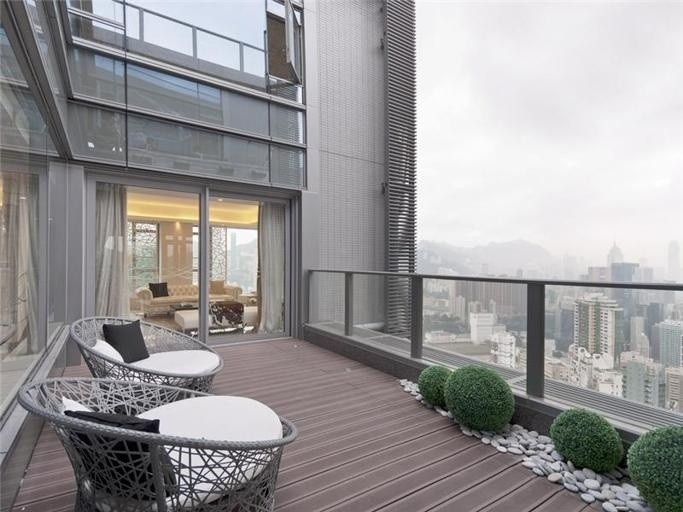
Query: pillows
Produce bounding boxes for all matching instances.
[64,410,177,501]
[91,339,123,363]
[209,280,225,294]
[61,395,89,415]
[149,282,169,297]
[102,319,149,364]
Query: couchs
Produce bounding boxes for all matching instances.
[135,283,242,318]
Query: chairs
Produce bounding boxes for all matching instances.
[18,378,297,512]
[70,316,223,392]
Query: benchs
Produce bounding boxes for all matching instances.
[175,306,258,333]
